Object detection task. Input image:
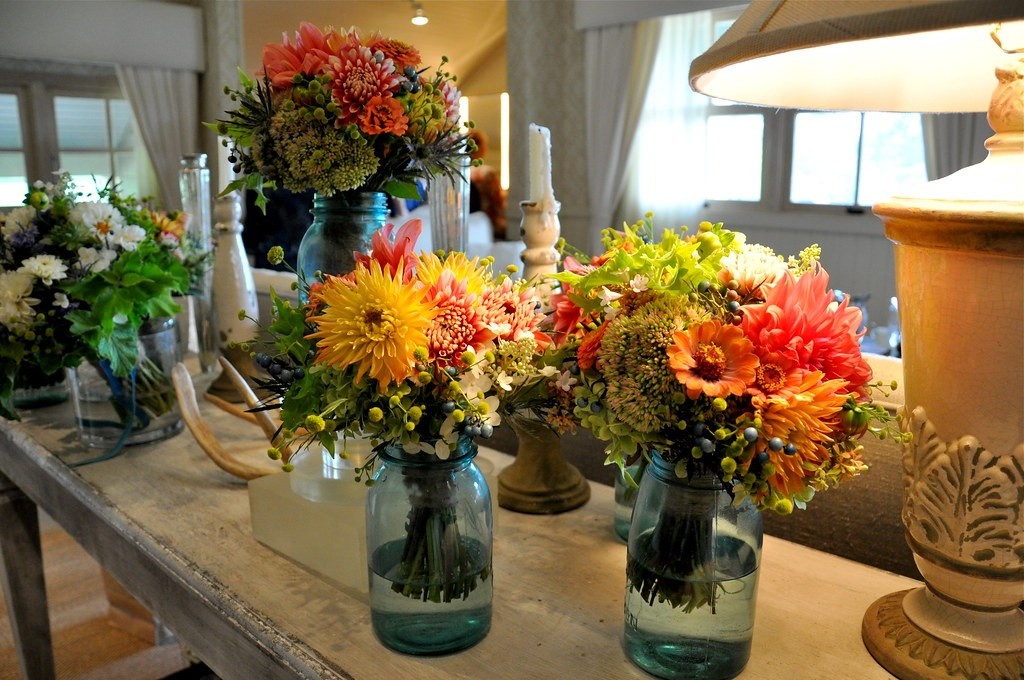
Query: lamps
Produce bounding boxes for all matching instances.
[684,1,1024,679]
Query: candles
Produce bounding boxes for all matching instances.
[218,136,236,193]
[529,121,554,204]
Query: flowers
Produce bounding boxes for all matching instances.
[238,218,555,491]
[200,21,484,216]
[493,266,583,437]
[546,212,652,492]
[1,171,210,377]
[547,219,913,516]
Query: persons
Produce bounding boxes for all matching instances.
[464,130,509,241]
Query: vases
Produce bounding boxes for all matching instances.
[497,407,591,514]
[298,189,391,309]
[69,314,189,453]
[365,442,496,659]
[619,452,764,680]
[612,442,650,543]
